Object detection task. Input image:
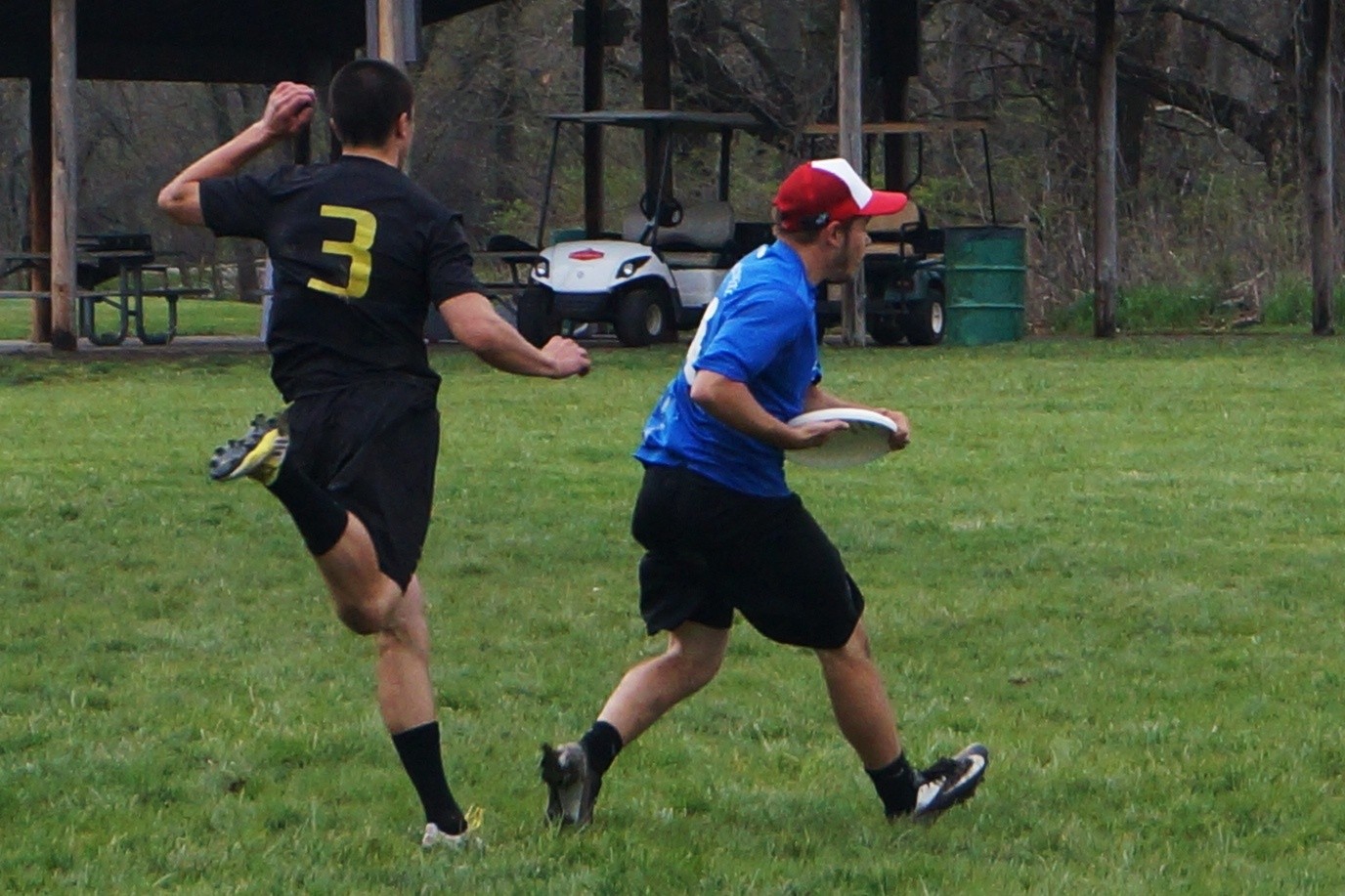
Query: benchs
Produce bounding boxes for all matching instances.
[0,287,212,301]
[861,201,948,273]
[484,287,522,301]
[623,198,736,266]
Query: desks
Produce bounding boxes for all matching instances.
[469,249,538,288]
[0,250,185,347]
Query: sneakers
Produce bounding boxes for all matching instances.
[539,741,602,838]
[422,804,490,858]
[208,408,291,485]
[887,743,988,830]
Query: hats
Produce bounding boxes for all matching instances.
[773,158,907,231]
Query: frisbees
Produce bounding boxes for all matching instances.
[782,408,897,474]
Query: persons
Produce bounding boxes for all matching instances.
[154,59,592,852]
[538,156,992,842]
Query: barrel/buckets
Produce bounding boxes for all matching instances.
[941,223,1028,347]
